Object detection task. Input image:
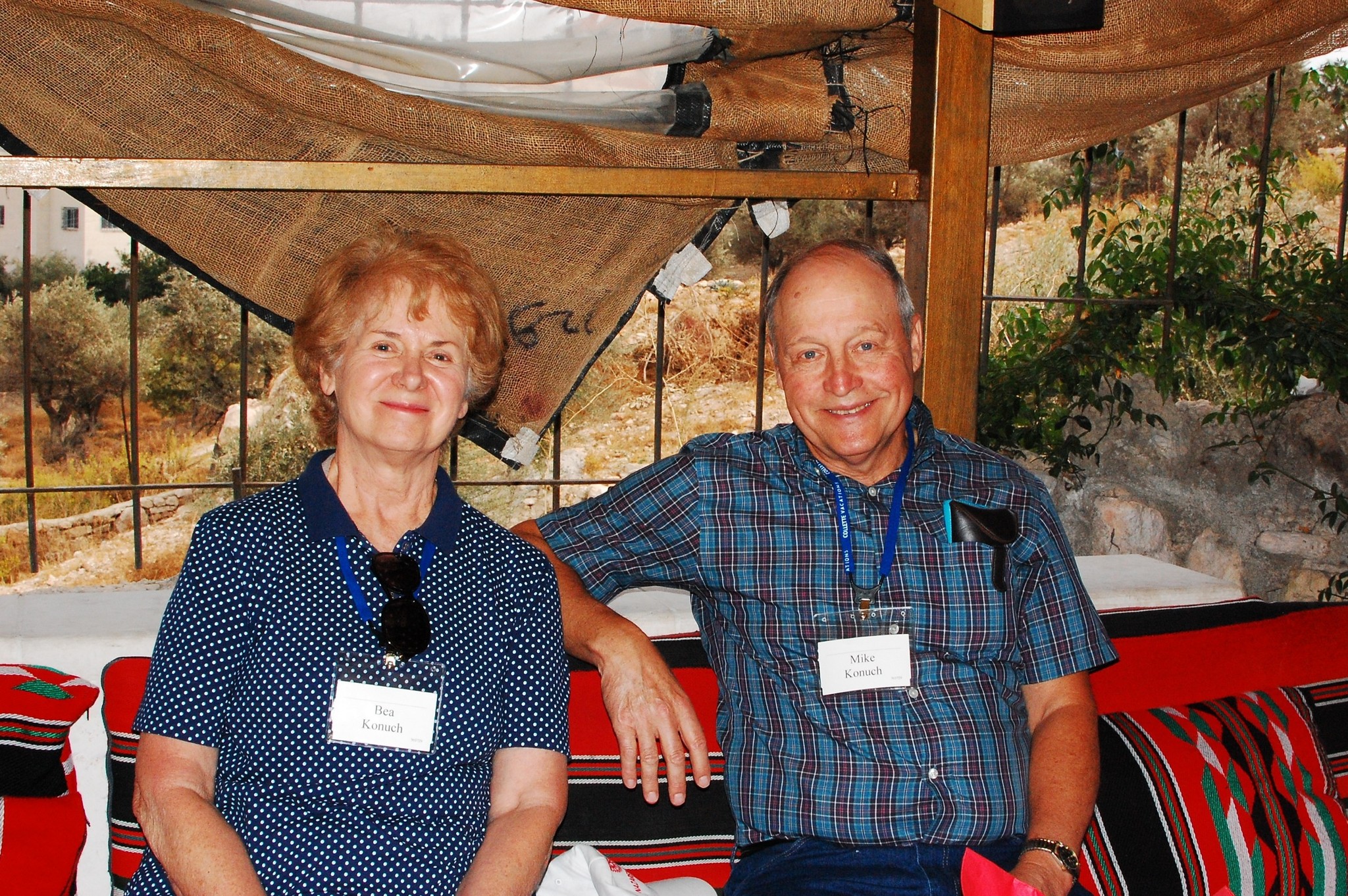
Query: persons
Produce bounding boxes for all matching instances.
[508,239,1120,896]
[120,227,573,896]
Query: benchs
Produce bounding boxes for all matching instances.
[1,596,1348,896]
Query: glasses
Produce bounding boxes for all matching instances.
[369,552,429,658]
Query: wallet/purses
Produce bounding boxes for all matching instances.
[949,500,1020,592]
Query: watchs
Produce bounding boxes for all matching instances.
[1018,838,1080,884]
